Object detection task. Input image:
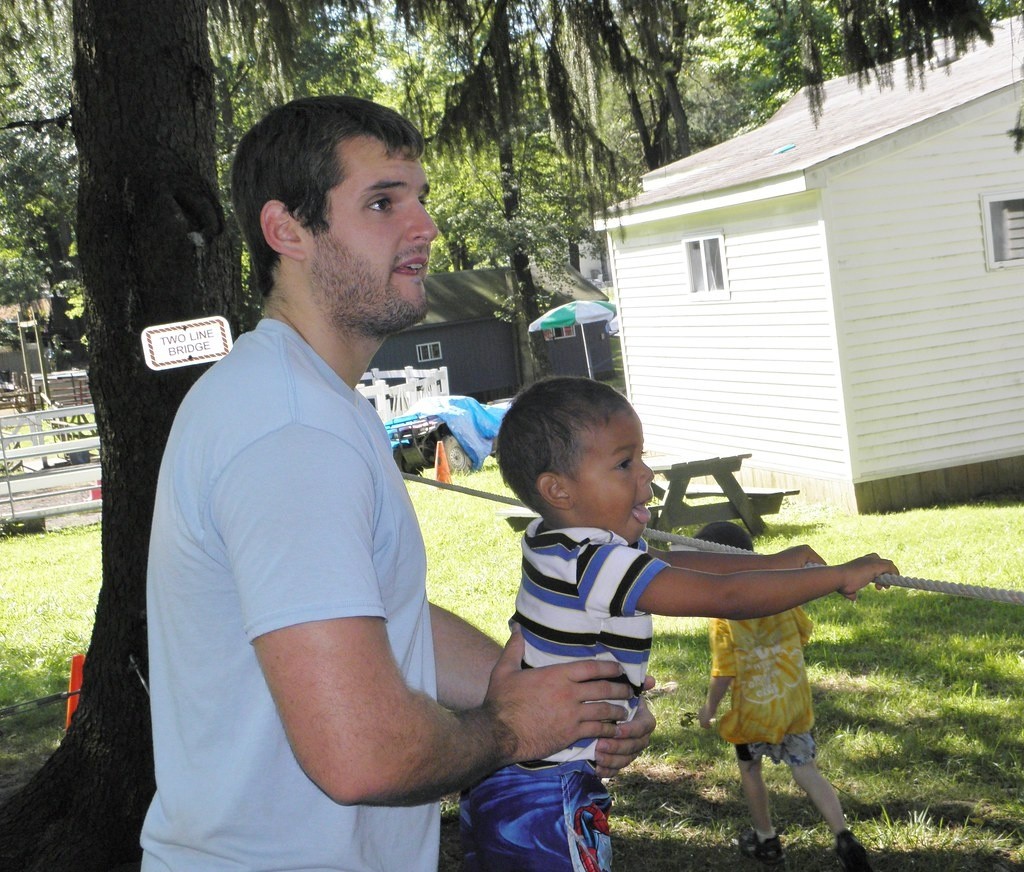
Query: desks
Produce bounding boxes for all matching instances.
[643,452,770,540]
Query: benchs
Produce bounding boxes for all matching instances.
[657,480,799,517]
[494,505,665,532]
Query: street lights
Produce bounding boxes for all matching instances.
[433,440,452,488]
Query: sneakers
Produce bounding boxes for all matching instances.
[739,829,786,866]
[835,830,873,872]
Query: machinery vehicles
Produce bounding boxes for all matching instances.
[386,396,501,478]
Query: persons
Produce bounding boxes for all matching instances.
[454,377,901,872]
[693,521,870,872]
[131,96,656,872]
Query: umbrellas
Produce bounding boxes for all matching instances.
[528,300,618,380]
